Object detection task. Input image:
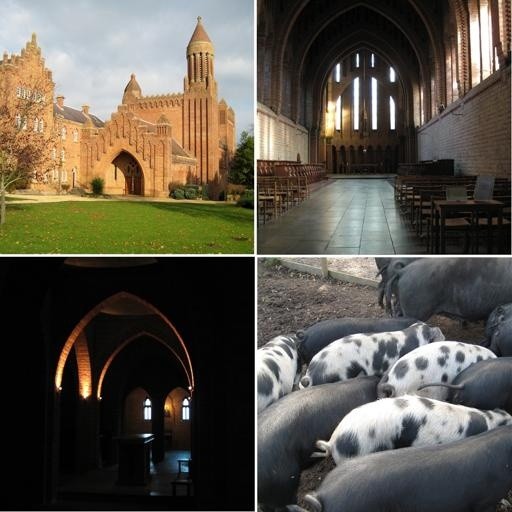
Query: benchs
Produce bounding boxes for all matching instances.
[257,175,307,225]
[395,173,509,254]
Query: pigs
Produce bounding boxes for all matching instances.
[258,259,511,508]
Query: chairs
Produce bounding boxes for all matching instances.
[171,458,192,496]
